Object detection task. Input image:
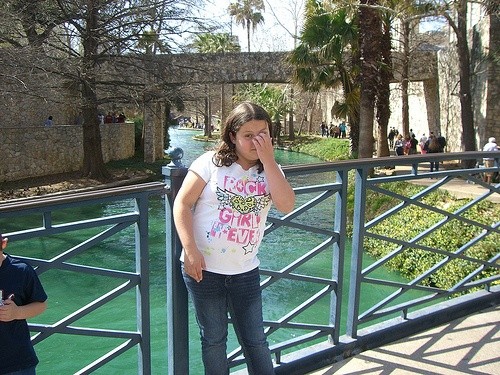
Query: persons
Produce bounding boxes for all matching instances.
[320,122,346,139]
[98,111,126,123]
[483,137,500,185]
[0,233,49,375]
[76,111,83,124]
[386,126,441,179]
[494,146,500,180]
[44,116,54,126]
[172,102,295,375]
